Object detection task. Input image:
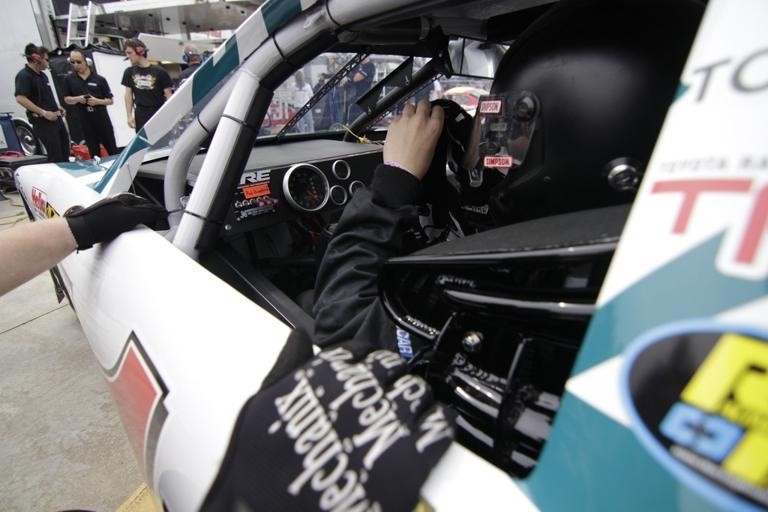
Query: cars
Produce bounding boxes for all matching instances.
[13,1,768,510]
[437,83,497,108]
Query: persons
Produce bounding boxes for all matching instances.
[0,189,171,301]
[15,43,71,164]
[306,3,670,367]
[175,45,202,92]
[120,37,172,137]
[289,71,314,133]
[61,48,119,160]
[350,52,375,98]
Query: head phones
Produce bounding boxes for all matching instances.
[182,54,202,63]
[67,57,93,66]
[136,46,149,55]
[23,53,41,64]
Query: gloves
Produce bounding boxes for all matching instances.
[63,188,172,252]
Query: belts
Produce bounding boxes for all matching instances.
[85,105,106,112]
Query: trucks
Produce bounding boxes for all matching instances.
[134,33,297,130]
[1,2,66,159]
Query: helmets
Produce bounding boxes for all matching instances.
[459,0,664,207]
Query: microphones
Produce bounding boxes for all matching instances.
[125,57,129,60]
